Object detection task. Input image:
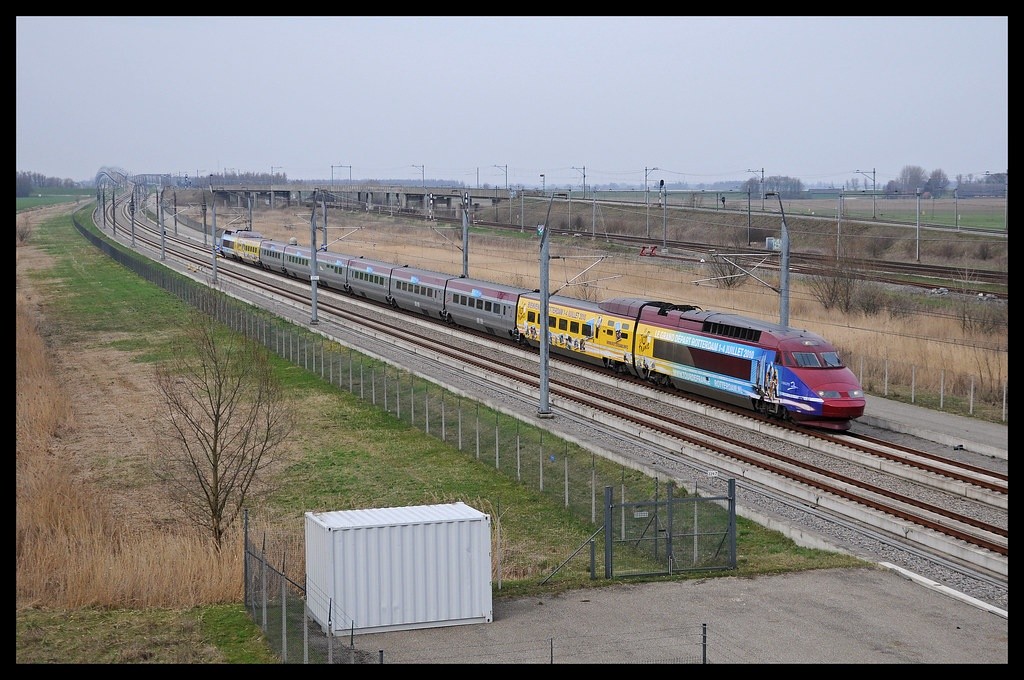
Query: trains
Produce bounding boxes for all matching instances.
[218,226,869,436]
